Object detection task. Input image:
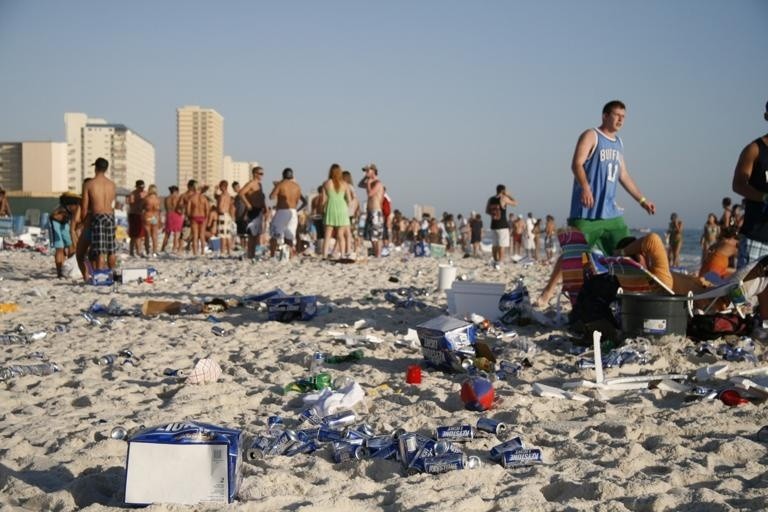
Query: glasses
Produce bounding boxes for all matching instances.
[255,172,264,175]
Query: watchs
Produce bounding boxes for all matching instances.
[640,197,646,205]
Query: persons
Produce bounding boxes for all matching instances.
[230,181,246,249]
[312,186,323,256]
[175,180,195,251]
[320,164,352,260]
[613,233,768,312]
[187,182,209,256]
[532,219,542,260]
[486,184,519,267]
[206,205,218,242]
[0,185,12,218]
[665,213,683,268]
[214,180,232,258]
[508,213,515,254]
[162,185,185,252]
[83,178,95,270]
[269,168,301,262]
[48,203,81,280]
[383,187,391,248]
[525,211,537,258]
[331,171,358,258]
[392,209,484,256]
[516,214,525,253]
[358,163,384,258]
[701,214,720,264]
[127,180,147,257]
[731,203,744,230]
[721,197,733,229]
[139,185,160,255]
[81,157,116,270]
[732,101,768,271]
[239,167,268,258]
[545,214,556,262]
[532,100,656,306]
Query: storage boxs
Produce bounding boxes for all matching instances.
[444,288,505,323]
[122,421,245,506]
[415,313,477,371]
[414,242,432,258]
[267,296,320,324]
[92,269,114,286]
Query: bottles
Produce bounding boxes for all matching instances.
[413,432,436,451]
[490,437,525,463]
[696,334,761,367]
[399,431,423,468]
[601,342,652,371]
[477,416,507,436]
[423,451,468,477]
[327,347,366,363]
[577,356,595,369]
[339,426,370,445]
[502,448,541,469]
[315,425,346,446]
[382,284,430,311]
[391,426,406,444]
[110,425,128,441]
[285,430,310,457]
[434,421,474,442]
[357,423,376,440]
[693,385,718,395]
[365,432,393,453]
[286,438,320,459]
[406,436,439,475]
[308,351,325,374]
[452,310,536,382]
[164,367,183,376]
[299,407,323,426]
[245,430,273,462]
[322,408,357,430]
[432,438,467,457]
[210,326,226,338]
[283,372,334,397]
[98,354,117,368]
[266,414,282,435]
[267,427,299,458]
[333,445,370,464]
[371,442,397,464]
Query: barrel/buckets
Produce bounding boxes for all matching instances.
[617,285,693,337]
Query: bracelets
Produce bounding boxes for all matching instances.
[639,197,647,204]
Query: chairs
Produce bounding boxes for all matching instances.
[553,231,624,330]
[596,256,742,328]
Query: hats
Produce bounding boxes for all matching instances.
[91,157,109,168]
[361,163,378,172]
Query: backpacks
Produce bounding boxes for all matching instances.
[60,191,82,213]
